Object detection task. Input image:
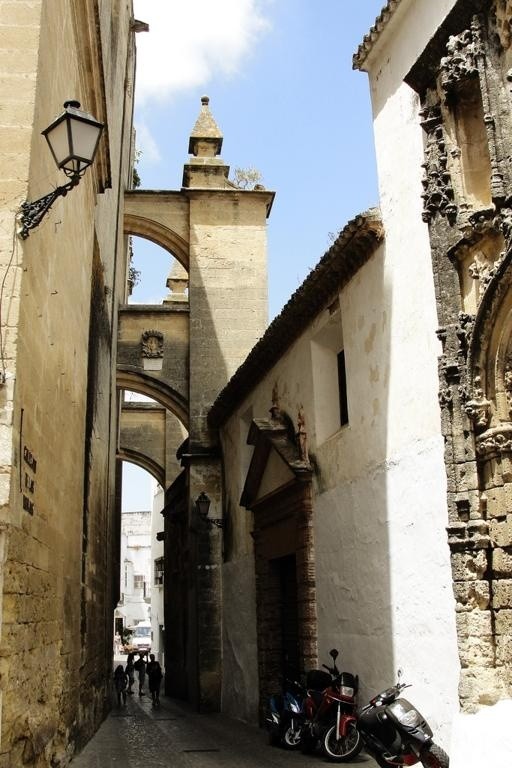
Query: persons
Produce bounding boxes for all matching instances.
[138,651,147,696]
[115,665,128,707]
[145,654,161,705]
[125,653,135,695]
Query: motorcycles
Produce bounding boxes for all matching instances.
[261,674,305,749]
[355,668,449,767]
[298,648,364,762]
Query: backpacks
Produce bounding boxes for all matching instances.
[134,661,139,672]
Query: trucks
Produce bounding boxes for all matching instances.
[131,621,152,653]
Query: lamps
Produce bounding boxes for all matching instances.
[16,100,106,240]
[195,491,224,528]
